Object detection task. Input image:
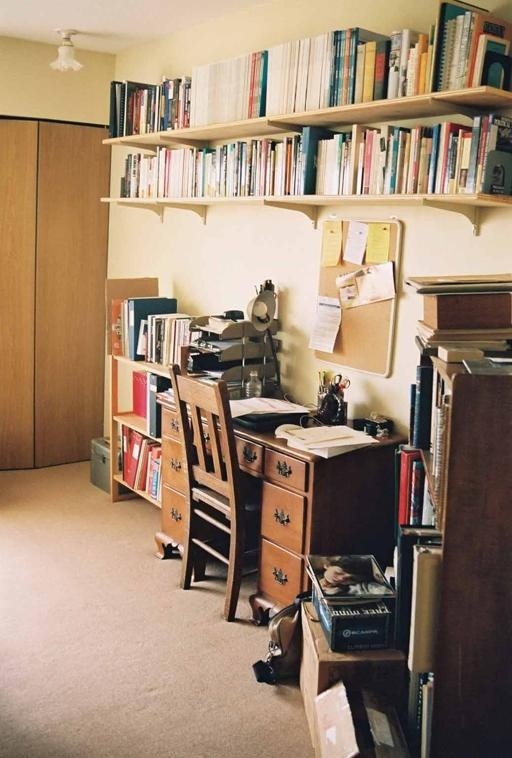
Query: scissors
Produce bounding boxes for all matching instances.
[334,374,350,393]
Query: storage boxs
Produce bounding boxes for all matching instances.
[297,601,406,749]
[90,436,110,496]
[315,681,409,757]
[312,580,394,652]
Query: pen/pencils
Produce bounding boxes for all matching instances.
[319,371,326,394]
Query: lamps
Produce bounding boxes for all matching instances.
[50,29,81,72]
[240,291,280,395]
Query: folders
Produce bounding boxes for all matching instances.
[121,297,177,362]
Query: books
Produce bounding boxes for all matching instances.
[306,553,396,599]
[112,294,207,503]
[403,271,512,352]
[287,435,371,459]
[394,364,453,757]
[111,1,509,137]
[305,566,382,605]
[118,114,510,195]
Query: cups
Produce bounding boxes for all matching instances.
[317,394,344,425]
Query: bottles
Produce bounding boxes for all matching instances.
[246,370,262,399]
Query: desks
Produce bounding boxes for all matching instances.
[155,392,410,627]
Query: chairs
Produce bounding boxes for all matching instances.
[168,364,261,622]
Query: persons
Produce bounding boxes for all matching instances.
[319,557,370,597]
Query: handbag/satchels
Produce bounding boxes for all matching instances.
[252,589,312,685]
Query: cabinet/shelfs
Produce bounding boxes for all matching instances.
[112,354,173,508]
[99,86,512,235]
[404,356,511,758]
